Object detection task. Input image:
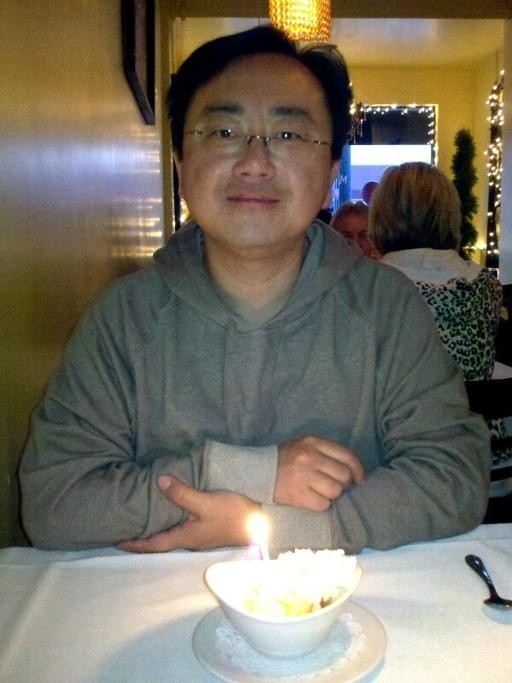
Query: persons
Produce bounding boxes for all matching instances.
[365,159,512,500]
[17,15,493,555]
[326,198,381,260]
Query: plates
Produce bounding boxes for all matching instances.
[192,599,388,683]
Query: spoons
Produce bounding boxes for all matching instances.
[464,554,512,610]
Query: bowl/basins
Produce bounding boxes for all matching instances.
[205,556,364,660]
